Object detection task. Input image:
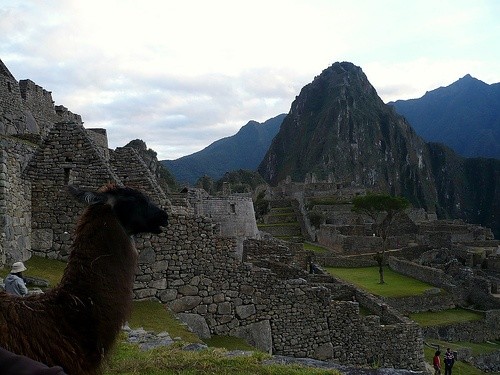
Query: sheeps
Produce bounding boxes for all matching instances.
[0,180,173,375]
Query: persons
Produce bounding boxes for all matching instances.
[443,348,457,375]
[5,262,28,296]
[309,266,314,273]
[433,350,442,375]
